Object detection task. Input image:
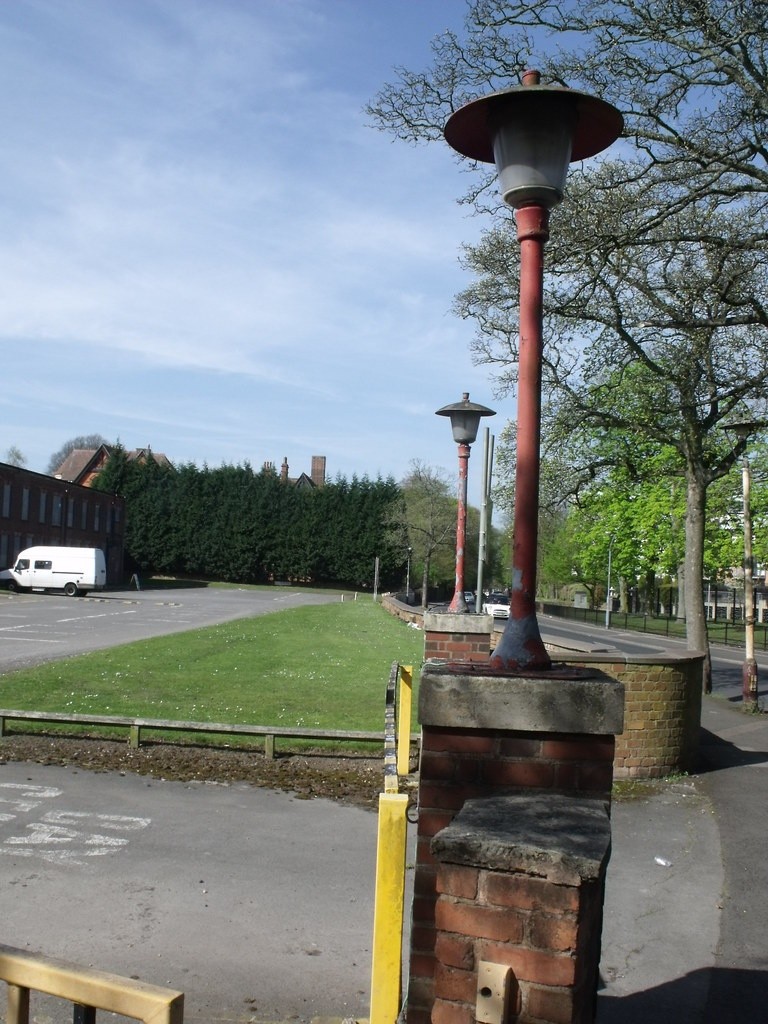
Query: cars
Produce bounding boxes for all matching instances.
[482,592,511,620]
[465,592,475,605]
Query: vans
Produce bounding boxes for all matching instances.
[0,545,106,598]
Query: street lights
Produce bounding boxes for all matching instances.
[406,547,411,606]
[720,413,768,715]
